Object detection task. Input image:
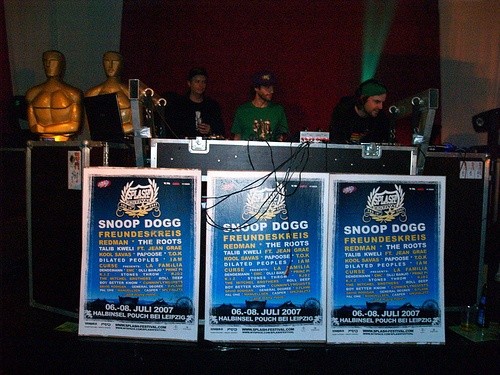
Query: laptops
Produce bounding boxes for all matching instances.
[83,92,133,142]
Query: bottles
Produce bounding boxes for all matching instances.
[477,288,490,328]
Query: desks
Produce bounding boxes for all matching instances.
[149,138,418,176]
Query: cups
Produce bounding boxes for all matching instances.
[460,305,475,330]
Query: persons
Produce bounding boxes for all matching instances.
[84,51,143,135]
[230,71,290,142]
[164,67,227,139]
[26,49,85,143]
[329,78,399,144]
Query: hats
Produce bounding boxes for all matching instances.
[359,79,388,97]
[252,72,275,88]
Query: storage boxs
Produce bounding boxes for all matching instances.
[425,146,492,316]
[24,141,127,319]
